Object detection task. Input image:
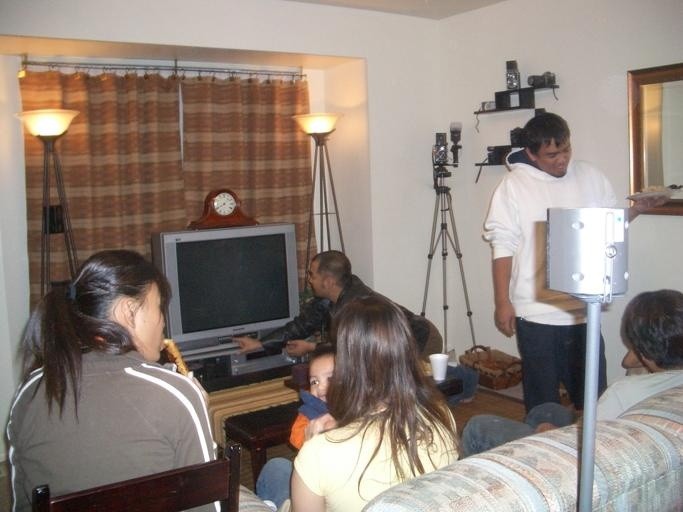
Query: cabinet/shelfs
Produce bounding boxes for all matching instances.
[176,346,306,394]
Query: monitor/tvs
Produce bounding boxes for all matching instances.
[149,221,301,364]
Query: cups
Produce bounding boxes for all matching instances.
[428,354,449,380]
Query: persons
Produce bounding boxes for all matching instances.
[289,349,338,448]
[232,251,480,404]
[6,249,222,512]
[482,112,619,413]
[458,288,682,460]
[256,294,461,512]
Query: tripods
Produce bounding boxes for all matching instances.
[419,163,478,354]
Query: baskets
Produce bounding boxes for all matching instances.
[458,345,524,390]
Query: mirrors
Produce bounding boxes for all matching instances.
[626,62,683,217]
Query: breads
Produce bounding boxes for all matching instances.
[162,338,188,377]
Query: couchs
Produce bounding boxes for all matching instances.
[213,383,682,512]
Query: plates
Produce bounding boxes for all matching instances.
[628,190,672,203]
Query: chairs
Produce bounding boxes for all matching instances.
[32,442,242,512]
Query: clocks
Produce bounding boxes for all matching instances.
[187,187,260,231]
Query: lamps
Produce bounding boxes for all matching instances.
[16,110,82,300]
[290,112,344,297]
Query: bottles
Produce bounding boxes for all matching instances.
[505,61,520,91]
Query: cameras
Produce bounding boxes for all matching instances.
[528,70,555,86]
